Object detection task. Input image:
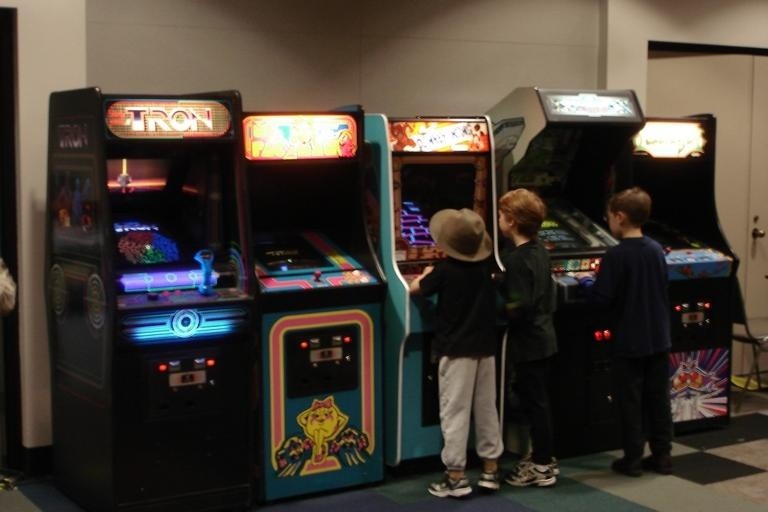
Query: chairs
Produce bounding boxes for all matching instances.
[721,266,767,415]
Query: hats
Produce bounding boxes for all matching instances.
[429,209,492,262]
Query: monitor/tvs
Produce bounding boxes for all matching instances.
[251,230,335,275]
[401,162,476,247]
[643,215,705,251]
[107,155,231,273]
[533,203,597,252]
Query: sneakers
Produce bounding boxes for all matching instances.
[477,469,501,490]
[429,476,471,498]
[612,456,642,477]
[504,454,559,488]
[643,455,673,474]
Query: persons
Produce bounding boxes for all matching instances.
[491,187,561,488]
[565,187,676,477]
[407,208,505,498]
